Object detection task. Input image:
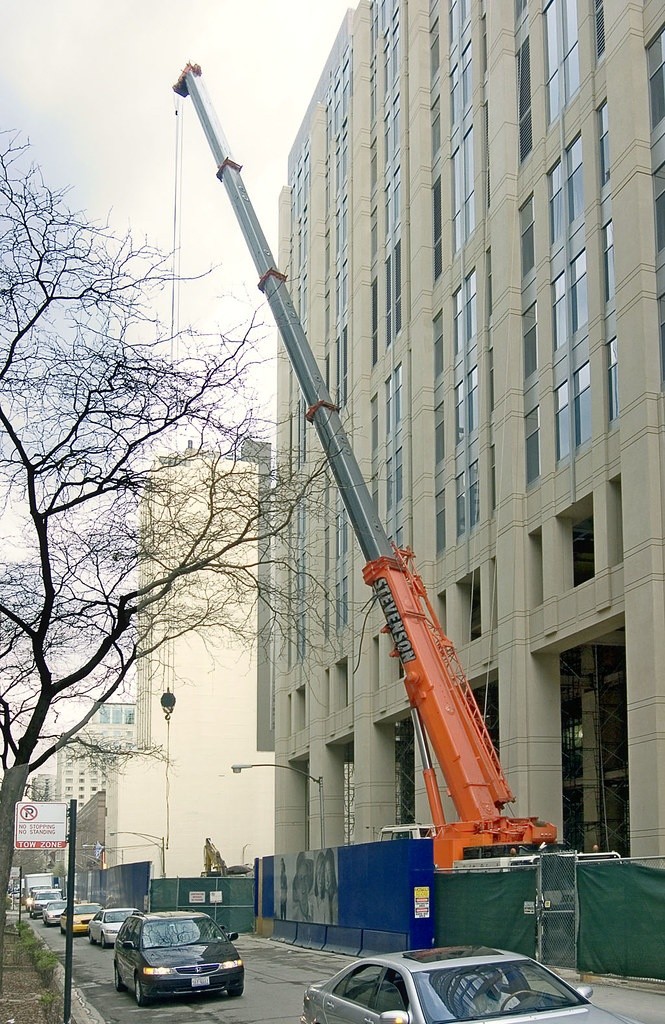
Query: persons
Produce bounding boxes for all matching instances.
[6,882,18,895]
[468,971,521,1017]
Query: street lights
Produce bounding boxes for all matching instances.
[231,764,325,850]
[109,831,166,878]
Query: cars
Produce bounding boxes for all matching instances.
[11,873,63,919]
[87,907,145,948]
[42,900,74,926]
[114,909,246,1006]
[60,902,107,935]
[299,943,651,1024]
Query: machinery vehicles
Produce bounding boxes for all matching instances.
[159,60,558,871]
